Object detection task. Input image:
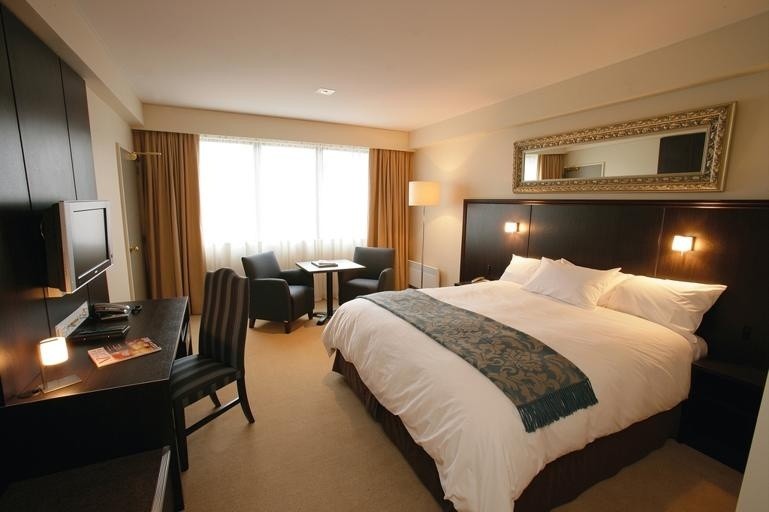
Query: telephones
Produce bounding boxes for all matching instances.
[471,277,490,284]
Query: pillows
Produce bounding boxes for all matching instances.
[521,256,632,311]
[499,254,540,285]
[598,275,728,343]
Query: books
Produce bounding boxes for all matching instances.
[318,259,336,265]
[88,336,162,368]
[311,261,338,268]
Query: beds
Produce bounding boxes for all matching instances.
[321,254,728,512]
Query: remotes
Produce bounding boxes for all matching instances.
[100,314,128,321]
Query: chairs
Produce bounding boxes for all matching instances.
[241,251,314,334]
[170,267,255,473]
[338,247,395,306]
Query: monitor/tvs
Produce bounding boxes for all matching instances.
[37,200,116,295]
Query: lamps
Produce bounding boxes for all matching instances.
[672,235,693,256]
[409,181,441,288]
[37,337,82,393]
[504,222,517,240]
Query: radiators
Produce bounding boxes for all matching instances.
[408,260,441,289]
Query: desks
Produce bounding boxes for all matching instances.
[0,296,193,512]
[295,260,367,326]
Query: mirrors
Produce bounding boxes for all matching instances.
[512,100,737,193]
[562,161,605,178]
